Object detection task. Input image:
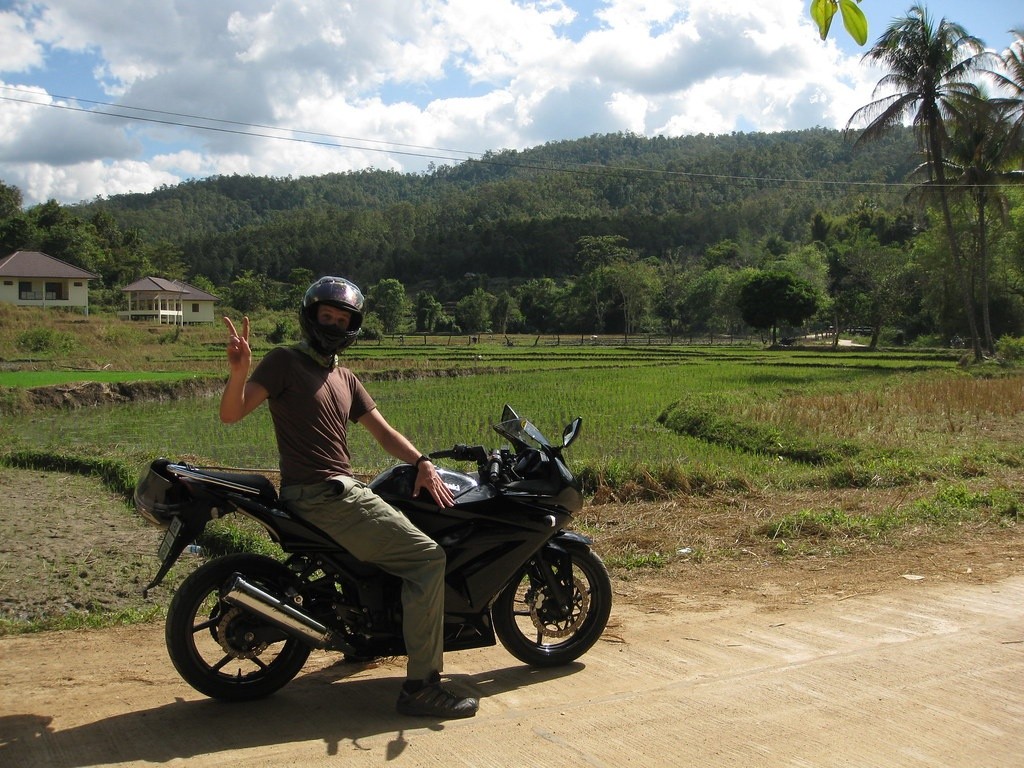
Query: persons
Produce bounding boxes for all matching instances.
[219,276,478,719]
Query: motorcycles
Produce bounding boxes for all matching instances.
[132,404,614,707]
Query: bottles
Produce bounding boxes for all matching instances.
[437,470,478,491]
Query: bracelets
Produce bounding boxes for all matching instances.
[416,454,431,470]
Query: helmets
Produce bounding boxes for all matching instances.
[298,275,366,353]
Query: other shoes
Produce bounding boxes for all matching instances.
[397,678,479,718]
